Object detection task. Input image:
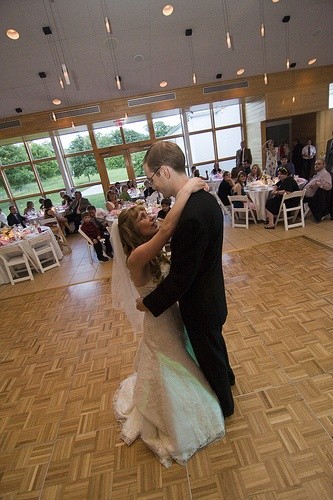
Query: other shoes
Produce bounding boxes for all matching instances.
[105,252,113,258]
[99,256,108,261]
[264,225,275,229]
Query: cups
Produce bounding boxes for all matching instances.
[110,186,162,218]
[246,174,299,190]
[27,204,66,217]
[0,219,38,244]
[210,169,225,180]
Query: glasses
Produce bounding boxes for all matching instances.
[136,211,151,223]
[148,167,161,187]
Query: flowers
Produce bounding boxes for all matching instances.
[135,199,145,204]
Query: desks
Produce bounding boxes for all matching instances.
[105,201,162,234]
[27,208,76,235]
[203,178,222,191]
[243,177,308,224]
[127,188,144,198]
[0,226,64,287]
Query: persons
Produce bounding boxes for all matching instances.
[210,162,222,174]
[62,195,73,209]
[103,181,136,212]
[217,160,262,219]
[39,197,45,209]
[144,179,156,199]
[110,205,225,469]
[236,140,252,168]
[24,201,34,215]
[6,206,27,229]
[81,211,113,261]
[135,140,236,416]
[0,207,7,228]
[157,198,172,219]
[190,165,209,180]
[60,191,75,205]
[87,205,110,239]
[44,199,74,237]
[65,191,91,236]
[260,129,333,229]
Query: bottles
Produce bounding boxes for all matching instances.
[156,194,161,208]
[261,170,267,180]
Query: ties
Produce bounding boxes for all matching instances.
[308,146,310,156]
[73,200,79,214]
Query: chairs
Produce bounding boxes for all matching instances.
[0,243,39,286]
[227,194,257,229]
[29,233,60,273]
[303,182,333,221]
[274,189,307,230]
[210,191,238,221]
[77,224,106,262]
[39,216,73,255]
